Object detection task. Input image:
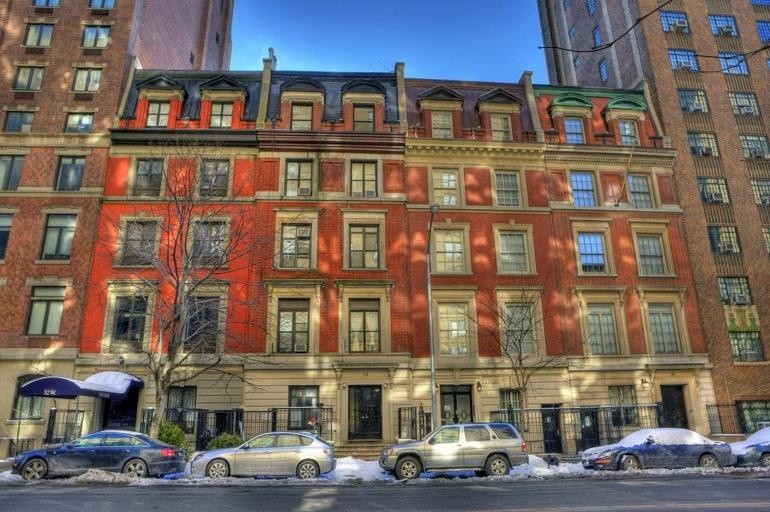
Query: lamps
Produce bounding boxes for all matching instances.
[642,378,648,387]
[342,381,482,392]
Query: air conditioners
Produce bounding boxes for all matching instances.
[674,19,770,305]
[296,227,310,238]
[298,187,311,196]
[293,342,307,352]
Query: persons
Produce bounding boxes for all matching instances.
[304,417,318,444]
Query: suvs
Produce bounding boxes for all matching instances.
[379,422,529,480]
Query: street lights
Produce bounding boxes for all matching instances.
[426,203,440,430]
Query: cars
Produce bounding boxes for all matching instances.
[581,428,738,471]
[190,432,334,479]
[728,426,770,467]
[12,429,187,482]
